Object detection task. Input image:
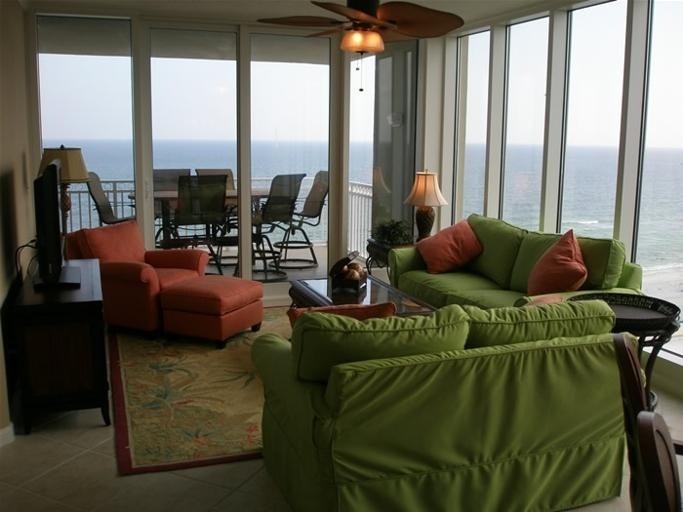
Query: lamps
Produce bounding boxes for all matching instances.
[340,23,384,90]
[36,144,89,235]
[401,169,449,243]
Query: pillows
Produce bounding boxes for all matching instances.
[419,220,483,274]
[525,228,587,296]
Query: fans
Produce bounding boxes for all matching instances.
[256,0,465,41]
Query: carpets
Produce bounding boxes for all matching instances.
[106,304,297,477]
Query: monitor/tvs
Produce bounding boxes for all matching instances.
[33,158,82,291]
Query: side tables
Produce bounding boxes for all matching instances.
[366,238,414,281]
[567,293,681,411]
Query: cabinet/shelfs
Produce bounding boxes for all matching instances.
[4,258,111,435]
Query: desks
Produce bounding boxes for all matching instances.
[128,187,269,272]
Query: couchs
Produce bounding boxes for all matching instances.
[249,300,647,512]
[387,213,643,313]
[65,219,264,348]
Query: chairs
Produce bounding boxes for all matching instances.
[226,173,307,283]
[86,171,136,226]
[611,333,682,512]
[195,168,264,266]
[269,170,329,269]
[153,173,228,275]
[153,168,191,249]
